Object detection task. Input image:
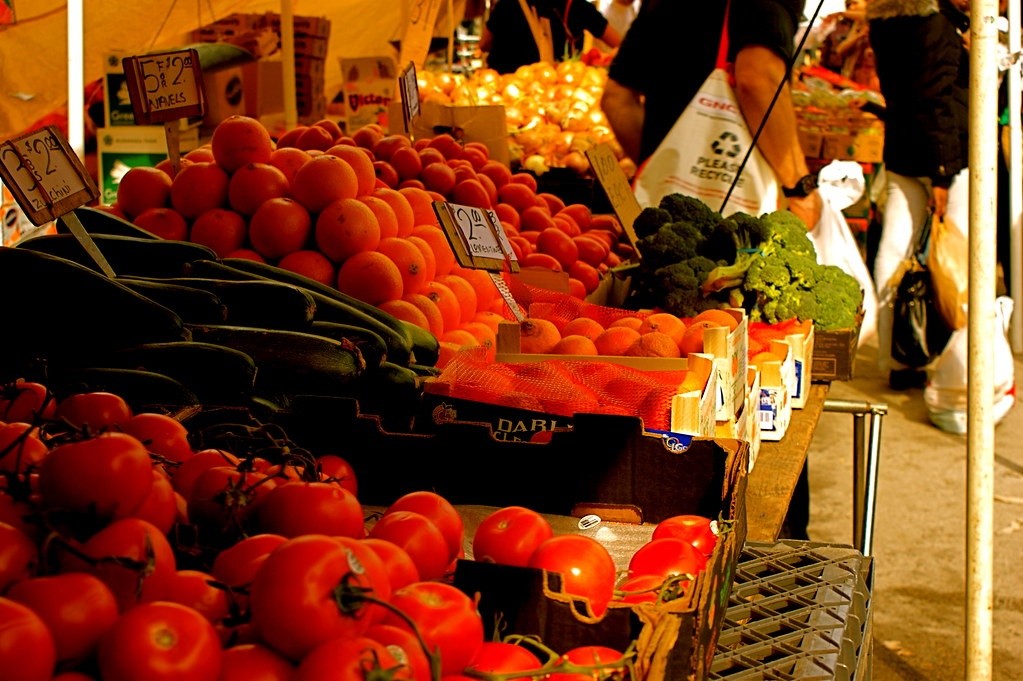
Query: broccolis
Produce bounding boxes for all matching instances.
[610,192,864,332]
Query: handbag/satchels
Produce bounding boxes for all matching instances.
[633,71,780,221]
[925,298,1018,434]
[890,251,952,365]
[930,212,968,325]
[806,159,876,349]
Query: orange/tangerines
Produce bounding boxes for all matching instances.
[92,114,739,430]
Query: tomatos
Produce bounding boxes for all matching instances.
[0,382,717,681]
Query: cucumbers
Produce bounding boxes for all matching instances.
[0,206,441,423]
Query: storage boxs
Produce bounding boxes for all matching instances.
[796,111,825,159]
[197,394,733,680]
[494,307,815,571]
[823,118,885,164]
[708,539,876,681]
[584,258,866,382]
[813,170,874,219]
[194,11,332,143]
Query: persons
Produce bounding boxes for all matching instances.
[864,0,1022,394]
[592,0,638,54]
[600,0,823,543]
[478,0,623,76]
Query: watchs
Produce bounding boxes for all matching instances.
[781,174,815,198]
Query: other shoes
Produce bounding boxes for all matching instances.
[890,369,928,391]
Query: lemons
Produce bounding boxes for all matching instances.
[410,62,638,182]
